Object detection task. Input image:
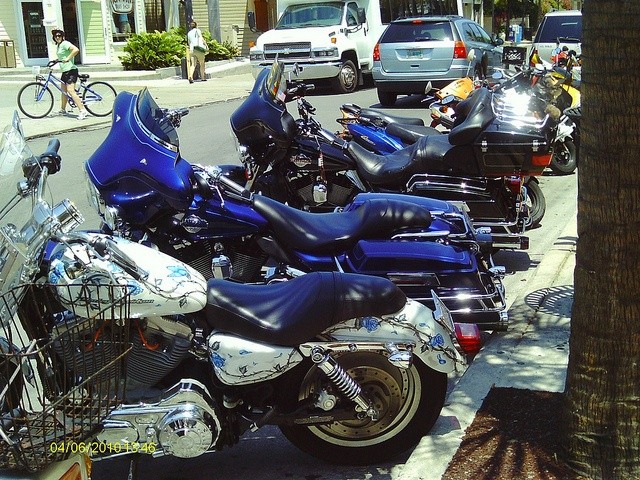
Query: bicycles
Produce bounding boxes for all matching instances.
[17,61,117,119]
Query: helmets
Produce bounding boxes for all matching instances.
[51,27,64,34]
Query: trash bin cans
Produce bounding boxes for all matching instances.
[0,39,17,68]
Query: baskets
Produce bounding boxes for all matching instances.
[33,65,50,81]
[0,282,139,474]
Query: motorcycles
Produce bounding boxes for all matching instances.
[0,138,467,465]
[429,105,576,175]
[83,89,510,349]
[339,80,441,126]
[336,117,546,230]
[421,49,581,132]
[217,52,562,246]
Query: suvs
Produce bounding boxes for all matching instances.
[531,8,583,64]
[371,15,504,106]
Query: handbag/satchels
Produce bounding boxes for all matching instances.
[194,35,205,53]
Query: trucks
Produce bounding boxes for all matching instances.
[248,0,463,93]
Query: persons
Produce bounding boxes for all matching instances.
[51,28,90,120]
[186,23,209,83]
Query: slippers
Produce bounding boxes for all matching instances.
[54,35,62,37]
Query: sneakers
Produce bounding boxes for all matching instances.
[53,108,68,115]
[77,108,90,120]
